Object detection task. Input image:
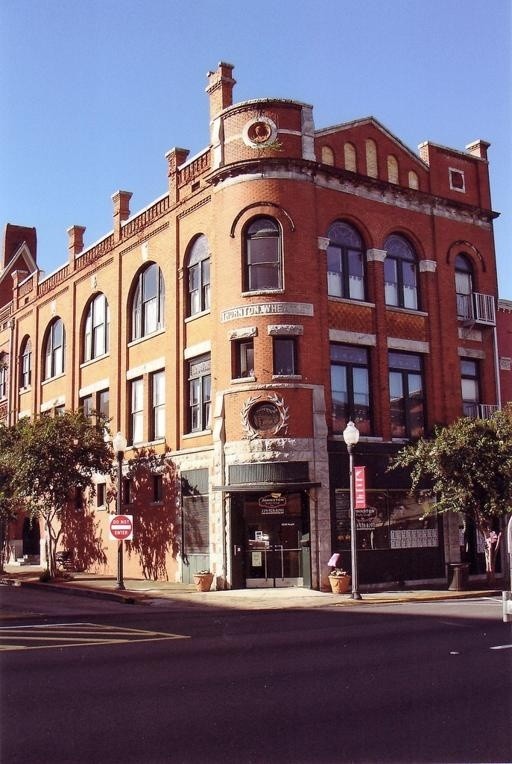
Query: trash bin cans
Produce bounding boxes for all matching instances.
[446,562,470,590]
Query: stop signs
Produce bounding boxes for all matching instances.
[108,515,133,541]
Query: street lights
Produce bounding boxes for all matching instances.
[341,420,367,602]
[110,429,129,591]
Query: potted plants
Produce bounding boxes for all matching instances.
[328,568,351,593]
[193,569,214,592]
